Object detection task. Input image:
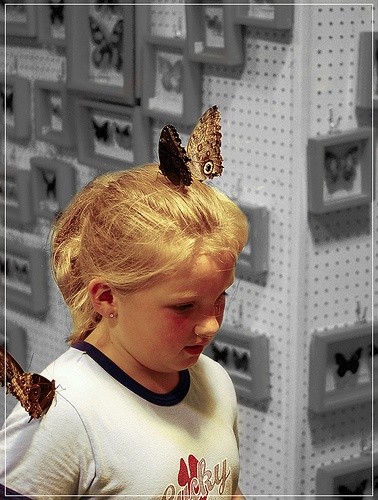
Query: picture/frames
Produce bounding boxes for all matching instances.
[63,0,137,108]
[306,124,375,215]
[141,34,207,127]
[184,0,246,68]
[37,1,67,48]
[0,72,31,143]
[229,198,269,276]
[0,319,28,373]
[306,322,378,414]
[0,236,54,315]
[232,0,294,31]
[201,324,271,402]
[316,453,378,500]
[0,164,36,232]
[73,100,153,175]
[0,0,38,39]
[356,31,378,109]
[34,79,78,149]
[30,157,77,220]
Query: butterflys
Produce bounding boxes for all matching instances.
[89,119,111,142]
[157,56,182,95]
[151,104,224,187]
[337,479,368,500]
[113,123,132,150]
[204,14,219,29]
[0,257,12,278]
[212,344,229,366]
[49,103,61,117]
[232,349,249,371]
[13,260,29,284]
[324,145,359,183]
[6,185,17,201]
[48,0,66,25]
[0,346,66,423]
[335,347,364,377]
[42,171,56,200]
[88,15,125,71]
[0,90,14,113]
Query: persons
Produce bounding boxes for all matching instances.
[0,162,248,500]
